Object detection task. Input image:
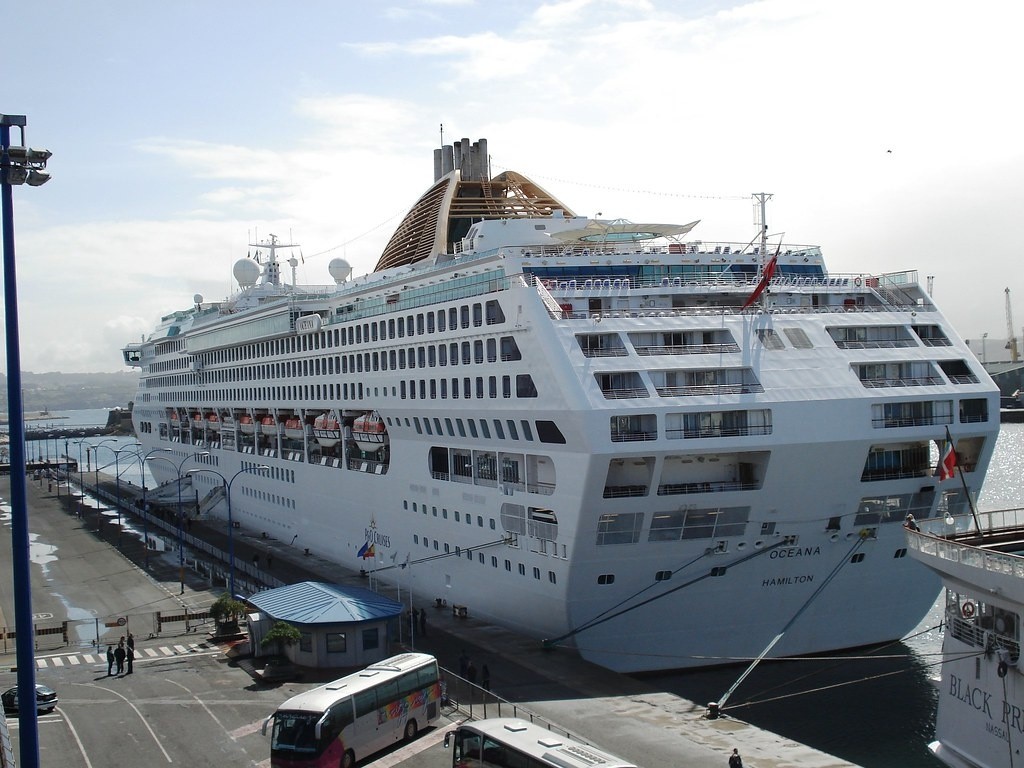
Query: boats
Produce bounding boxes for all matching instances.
[902,427,1024,768]
[171,411,389,453]
[120,124,1001,673]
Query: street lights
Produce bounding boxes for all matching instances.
[48,431,85,499]
[0,114,54,766]
[114,448,172,567]
[90,442,142,547]
[188,466,269,600]
[72,439,118,535]
[145,451,209,594]
[60,433,100,519]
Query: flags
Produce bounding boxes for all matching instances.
[939,429,957,483]
[740,241,781,311]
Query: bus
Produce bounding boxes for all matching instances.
[444,717,638,768]
[261,652,441,768]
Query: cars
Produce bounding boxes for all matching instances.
[1,684,59,715]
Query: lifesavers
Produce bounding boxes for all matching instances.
[543,280,553,290]
[962,602,974,616]
[997,662,1007,677]
[855,277,861,286]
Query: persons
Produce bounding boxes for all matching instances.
[107,646,114,676]
[195,501,200,518]
[460,649,477,685]
[145,501,152,513]
[127,632,134,649]
[114,643,126,674]
[161,479,168,486]
[1012,389,1020,397]
[252,550,260,567]
[983,619,999,649]
[729,748,743,768]
[125,645,134,675]
[135,497,144,508]
[481,664,491,695]
[118,636,125,648]
[407,606,426,636]
[174,511,192,532]
[903,513,920,533]
